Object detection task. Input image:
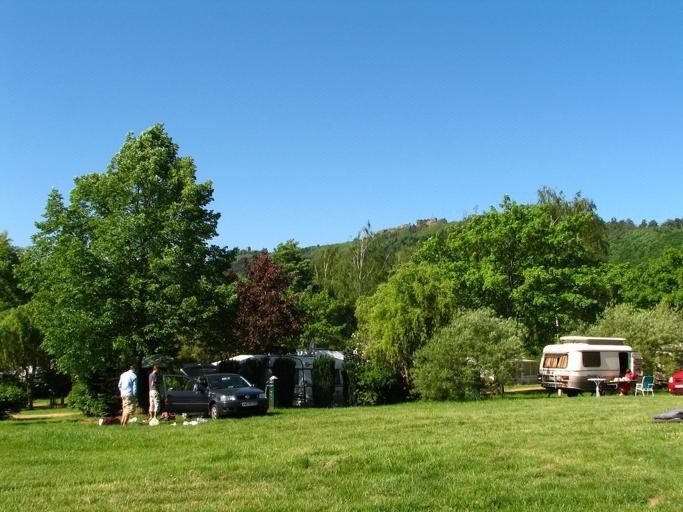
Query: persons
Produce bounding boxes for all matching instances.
[146,364,162,421]
[618,368,636,396]
[117,364,139,426]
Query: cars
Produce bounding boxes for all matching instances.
[668,369,683,394]
[163,373,268,418]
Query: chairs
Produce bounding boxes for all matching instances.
[635,376,654,397]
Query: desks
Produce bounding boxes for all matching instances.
[610,380,638,396]
[587,377,608,398]
